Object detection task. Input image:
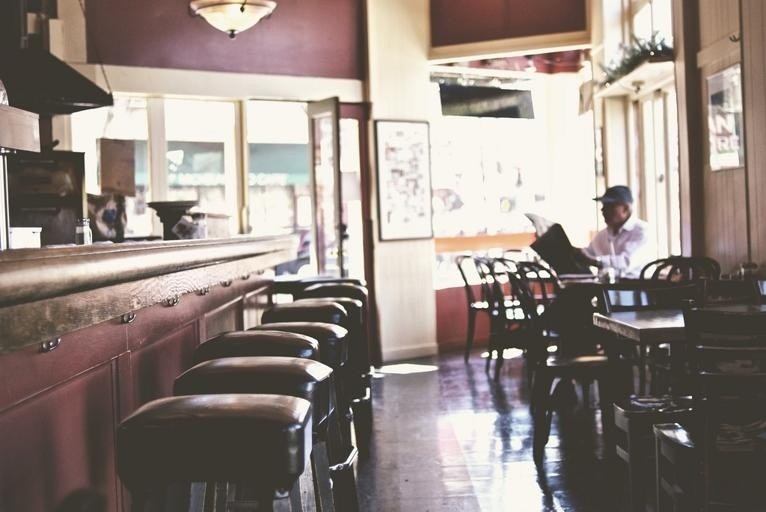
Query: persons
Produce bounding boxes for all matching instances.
[507,184,661,369]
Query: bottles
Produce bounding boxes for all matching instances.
[75,218,92,246]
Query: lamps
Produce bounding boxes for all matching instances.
[186,0,277,41]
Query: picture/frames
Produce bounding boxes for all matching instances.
[371,119,435,243]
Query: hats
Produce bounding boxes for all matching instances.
[592,186,633,204]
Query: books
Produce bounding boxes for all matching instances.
[523,209,593,274]
